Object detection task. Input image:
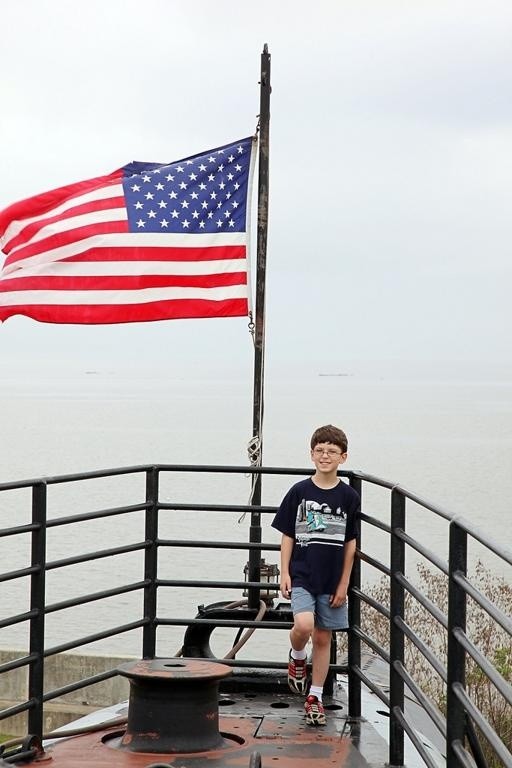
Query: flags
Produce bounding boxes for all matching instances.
[0,129,262,329]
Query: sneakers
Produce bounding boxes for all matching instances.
[304,696,328,726]
[287,647,310,694]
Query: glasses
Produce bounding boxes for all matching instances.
[313,448,344,458]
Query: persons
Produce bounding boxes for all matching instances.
[270,424,363,727]
